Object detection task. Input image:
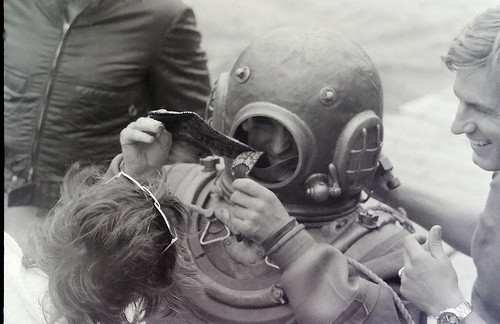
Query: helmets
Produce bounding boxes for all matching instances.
[204,28,383,228]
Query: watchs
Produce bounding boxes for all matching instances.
[435,301,473,324]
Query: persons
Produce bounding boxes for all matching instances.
[4,1,216,242]
[4,161,211,324]
[99,27,429,324]
[363,4,500,324]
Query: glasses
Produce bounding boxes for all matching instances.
[105,171,178,256]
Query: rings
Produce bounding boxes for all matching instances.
[398,267,405,278]
